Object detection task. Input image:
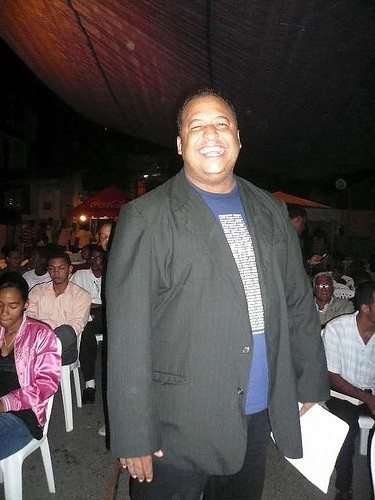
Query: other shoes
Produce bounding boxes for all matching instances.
[335,489,353,500]
[83,379,97,403]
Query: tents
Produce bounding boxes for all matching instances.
[271,190,333,208]
[70,186,137,222]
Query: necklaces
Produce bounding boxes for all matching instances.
[3,336,15,350]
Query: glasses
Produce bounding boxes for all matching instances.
[100,235,113,241]
[314,284,330,289]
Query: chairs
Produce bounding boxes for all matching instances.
[59,332,83,433]
[0,393,56,500]
[0,250,104,346]
[319,263,375,500]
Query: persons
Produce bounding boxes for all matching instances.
[104,87,332,500]
[22,252,91,365]
[0,272,63,461]
[286,204,329,275]
[313,272,355,329]
[306,247,375,290]
[0,217,115,292]
[320,279,375,500]
[67,247,106,404]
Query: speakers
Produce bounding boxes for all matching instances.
[4,189,23,210]
